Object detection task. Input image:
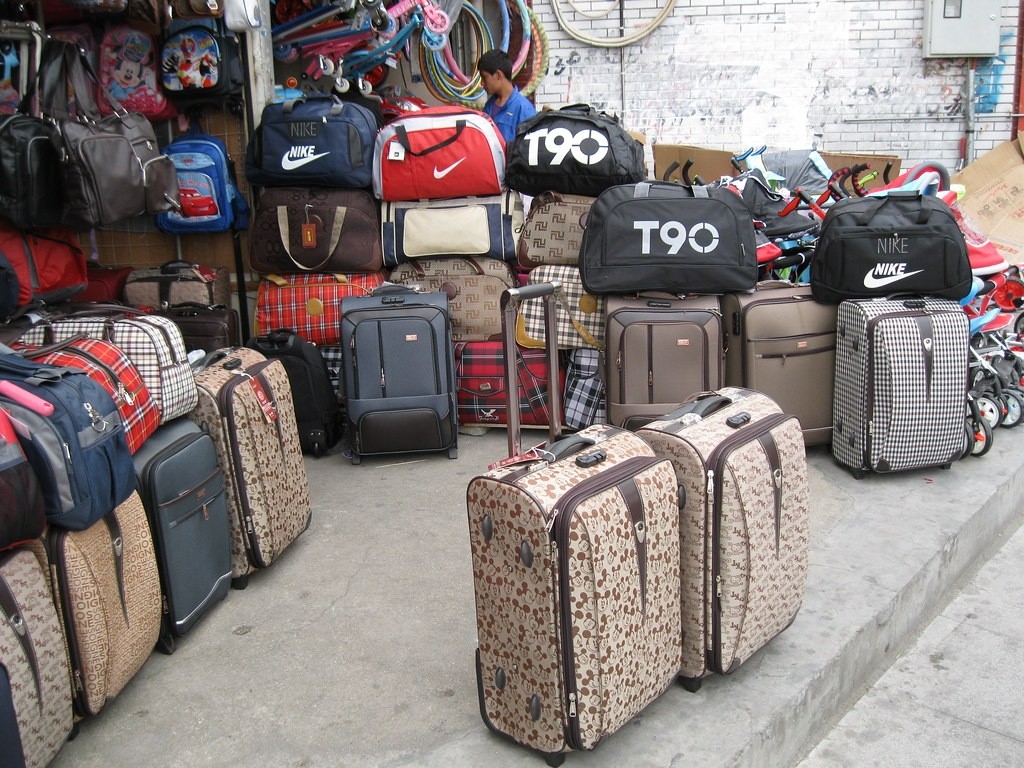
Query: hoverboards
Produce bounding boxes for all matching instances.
[271,0,449,96]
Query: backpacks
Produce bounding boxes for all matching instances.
[95,0,247,133]
[246,328,345,456]
[152,115,253,232]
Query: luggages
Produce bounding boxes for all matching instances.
[606,279,971,480]
[339,282,460,465]
[149,300,241,356]
[635,385,811,692]
[0,487,163,768]
[467,424,684,768]
[132,417,232,636]
[124,258,232,310]
[186,345,313,590]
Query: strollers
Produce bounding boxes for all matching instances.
[664,144,1024,459]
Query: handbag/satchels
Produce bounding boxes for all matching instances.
[579,180,758,296]
[564,346,611,430]
[451,340,564,426]
[247,93,648,345]
[46,42,183,231]
[809,190,973,305]
[0,38,69,230]
[0,226,198,554]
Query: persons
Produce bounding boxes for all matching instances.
[477,48,537,140]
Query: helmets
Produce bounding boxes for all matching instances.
[382,95,430,119]
